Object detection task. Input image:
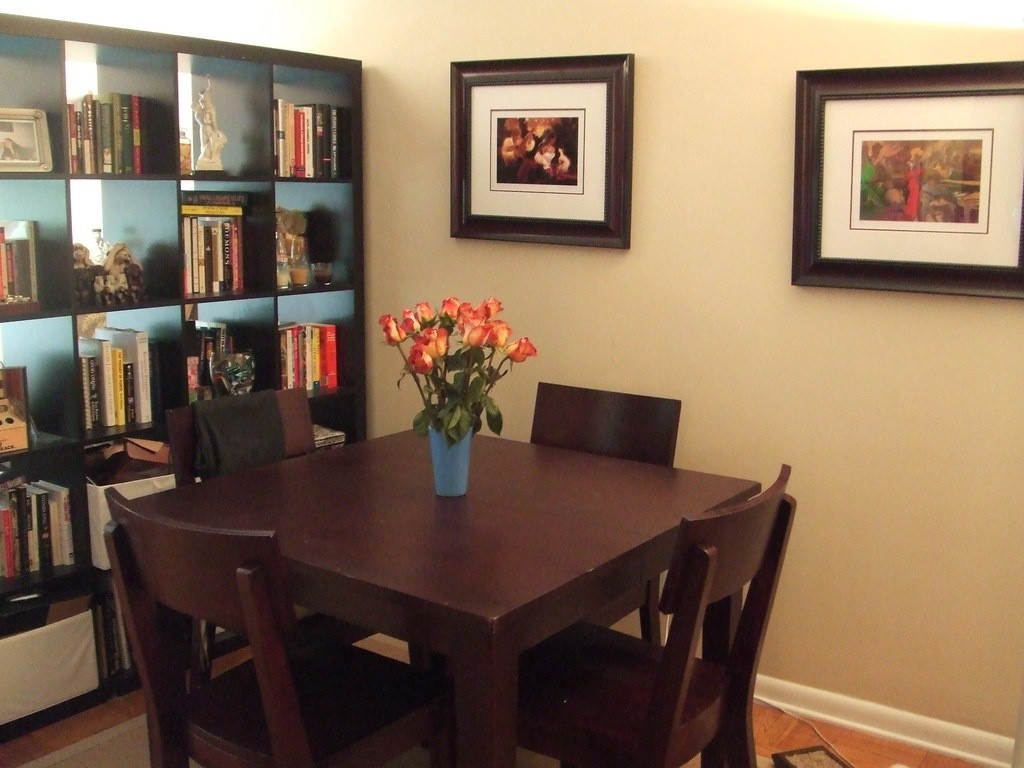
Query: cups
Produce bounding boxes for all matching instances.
[209,348,255,398]
[311,263,332,286]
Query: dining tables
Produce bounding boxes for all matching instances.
[124,427,763,768]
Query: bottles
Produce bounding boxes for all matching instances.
[276,231,289,289]
[289,237,309,289]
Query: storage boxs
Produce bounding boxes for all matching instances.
[0,608,99,727]
[83,437,176,572]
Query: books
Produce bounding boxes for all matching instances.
[0,90,354,592]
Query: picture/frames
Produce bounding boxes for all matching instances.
[450,51,636,251]
[0,108,53,173]
[790,58,1024,302]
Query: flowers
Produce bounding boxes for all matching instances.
[378,292,539,450]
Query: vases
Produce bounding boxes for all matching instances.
[428,423,473,497]
[771,746,853,768]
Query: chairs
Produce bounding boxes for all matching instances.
[515,463,798,768]
[530,381,683,642]
[102,485,458,767]
[168,386,366,699]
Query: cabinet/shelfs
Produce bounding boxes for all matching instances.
[0,13,371,727]
